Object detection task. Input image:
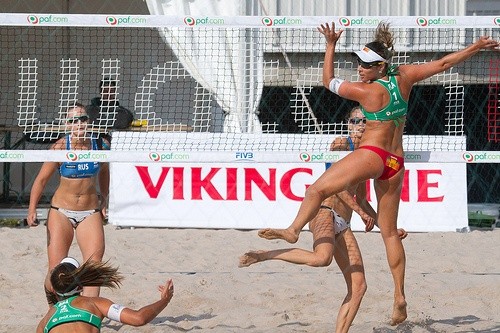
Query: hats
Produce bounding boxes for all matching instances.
[352,46,392,64]
[50,256,83,297]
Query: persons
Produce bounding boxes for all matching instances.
[36,261,173,333]
[259,20,500,332]
[27,102,110,301]
[239,108,408,333]
[90,76,135,223]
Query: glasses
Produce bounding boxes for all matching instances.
[357,57,381,69]
[67,115,90,124]
[350,117,366,124]
